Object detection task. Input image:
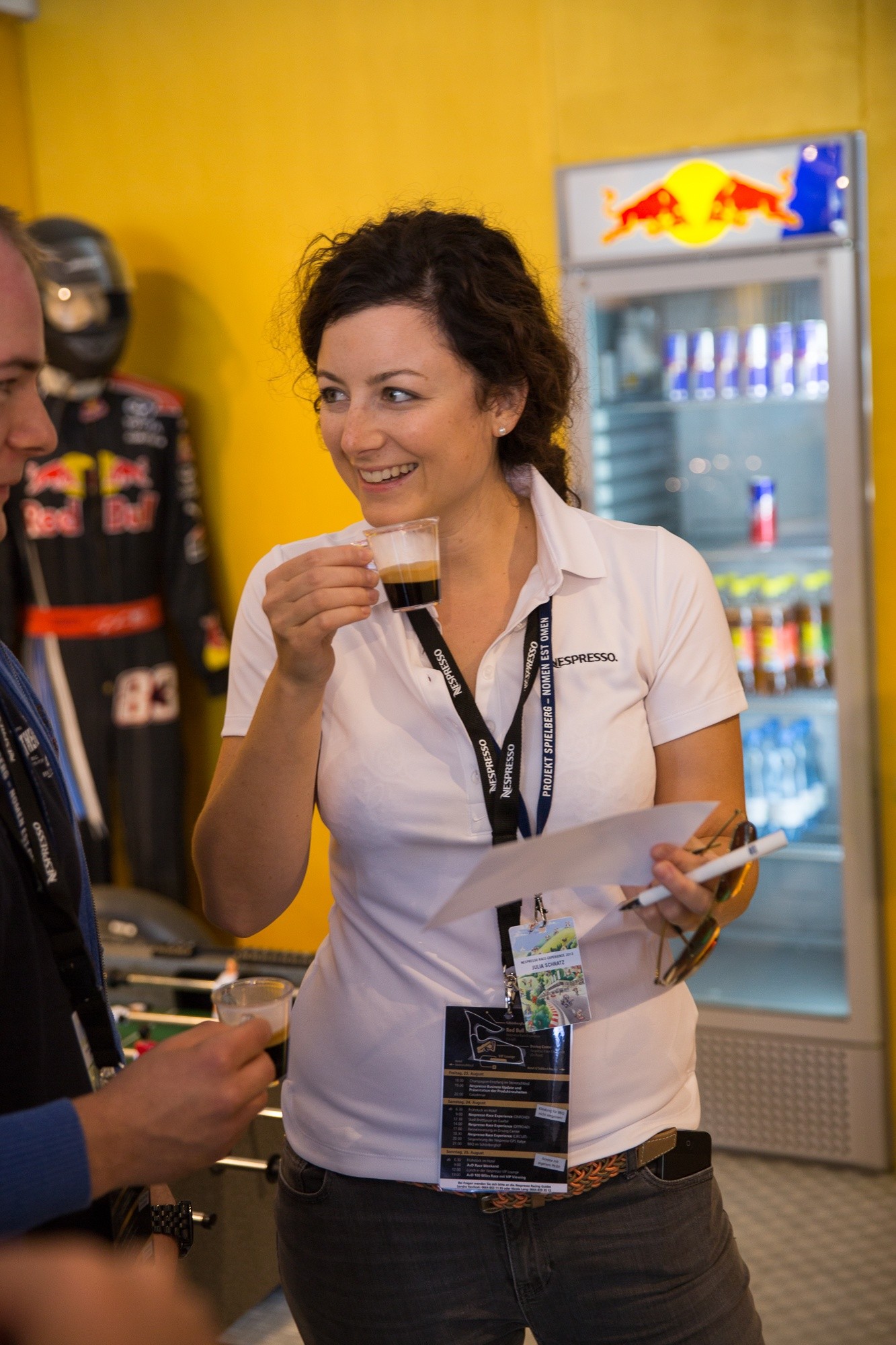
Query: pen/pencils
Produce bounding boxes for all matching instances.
[619,829,789,912]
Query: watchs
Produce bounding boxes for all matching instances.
[150,1200,194,1258]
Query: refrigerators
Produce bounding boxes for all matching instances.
[554,129,893,1173]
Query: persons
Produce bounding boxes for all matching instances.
[193,211,765,1345]
[0,205,277,1345]
[14,218,232,908]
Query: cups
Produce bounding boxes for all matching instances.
[211,976,294,1088]
[350,516,443,613]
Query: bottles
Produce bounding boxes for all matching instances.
[741,716,830,846]
[711,569,833,698]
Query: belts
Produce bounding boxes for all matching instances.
[415,1127,679,1213]
